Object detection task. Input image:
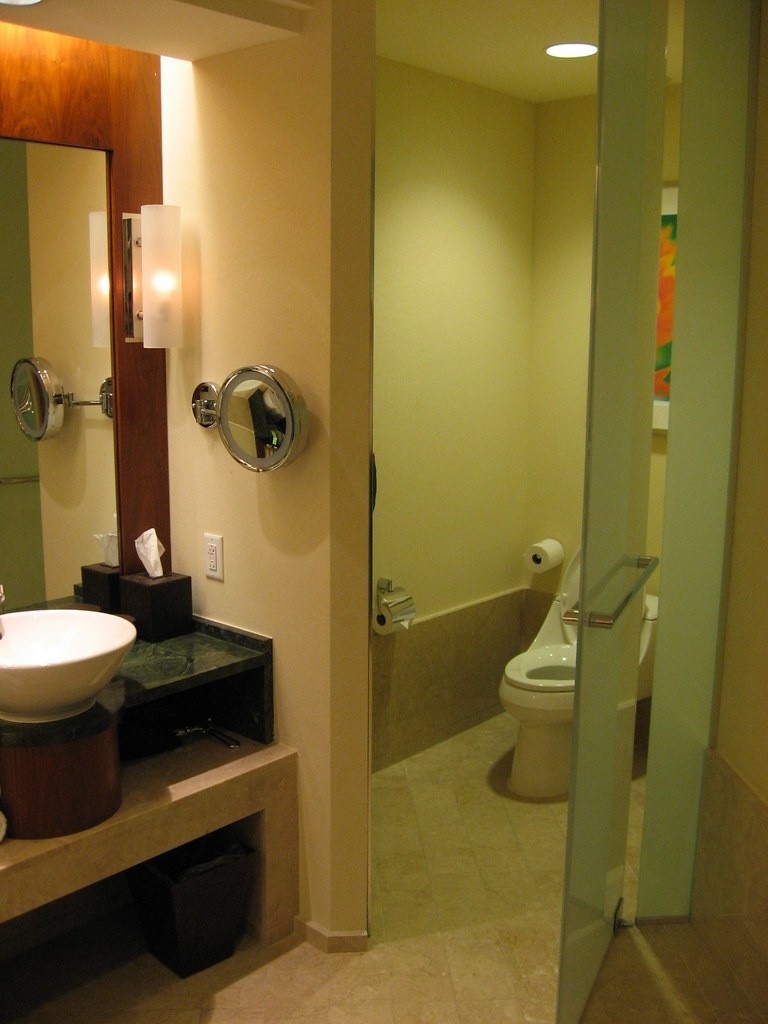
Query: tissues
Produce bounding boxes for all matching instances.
[118,527,196,644]
[80,527,120,616]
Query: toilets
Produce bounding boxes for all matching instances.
[494,542,660,801]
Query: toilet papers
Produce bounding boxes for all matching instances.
[522,537,566,575]
[371,587,417,636]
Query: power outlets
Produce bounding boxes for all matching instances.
[204,533,224,582]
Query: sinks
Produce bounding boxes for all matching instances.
[0,607,138,725]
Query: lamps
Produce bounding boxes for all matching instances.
[87,211,110,347]
[121,203,185,350]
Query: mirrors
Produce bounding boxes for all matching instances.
[11,358,64,443]
[0,135,125,611]
[214,362,309,474]
